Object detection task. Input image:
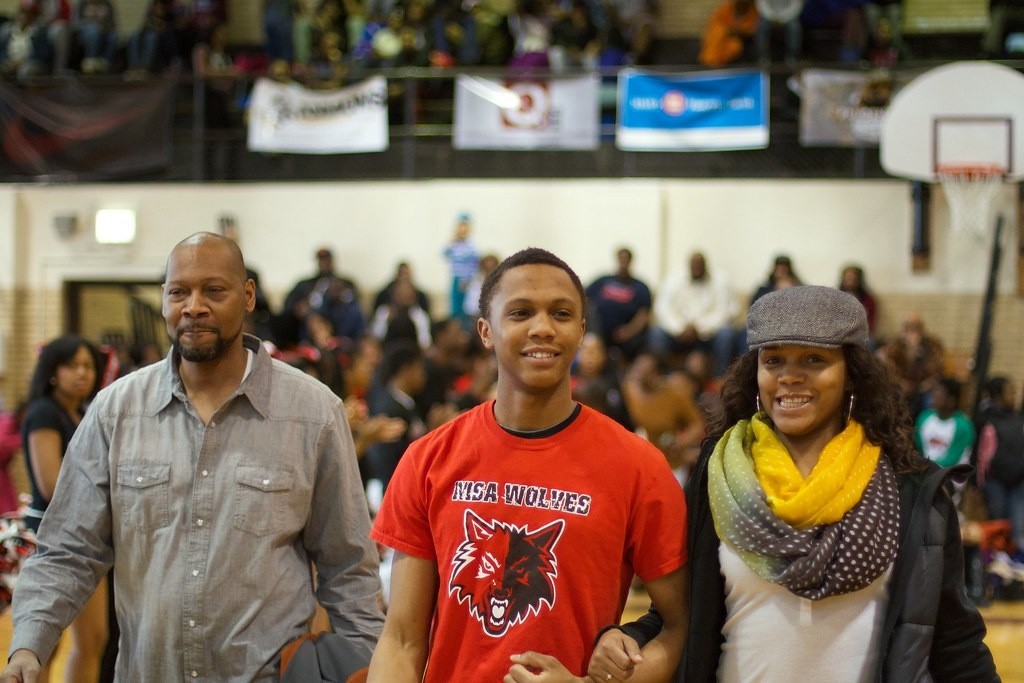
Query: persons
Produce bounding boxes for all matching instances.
[367,247,690,683]
[0,330,163,683]
[219,212,498,606]
[0,233,387,683]
[570,246,1024,602]
[587,282,1005,683]
[0,0,1024,179]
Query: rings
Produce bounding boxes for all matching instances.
[606,674,612,680]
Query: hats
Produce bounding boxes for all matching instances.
[747,285,869,350]
